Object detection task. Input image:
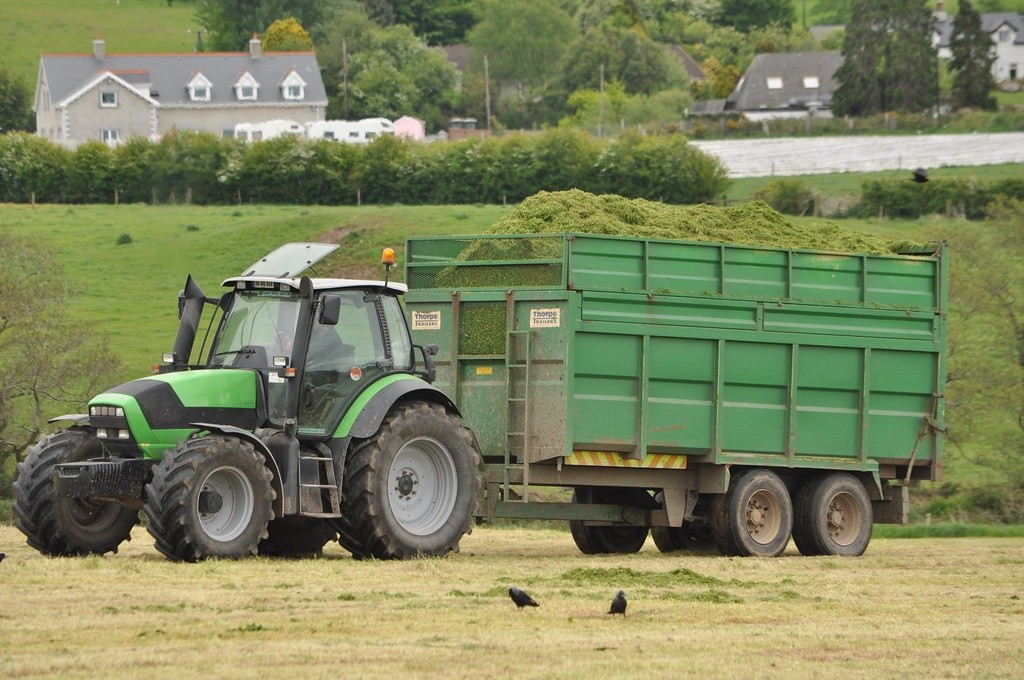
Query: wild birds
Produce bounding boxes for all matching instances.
[0,552,9,564]
[508,587,540,611]
[605,590,628,618]
[904,167,931,184]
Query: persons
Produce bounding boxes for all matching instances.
[276,312,343,370]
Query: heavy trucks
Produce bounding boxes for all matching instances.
[8,221,950,565]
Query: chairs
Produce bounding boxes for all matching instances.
[318,345,356,360]
